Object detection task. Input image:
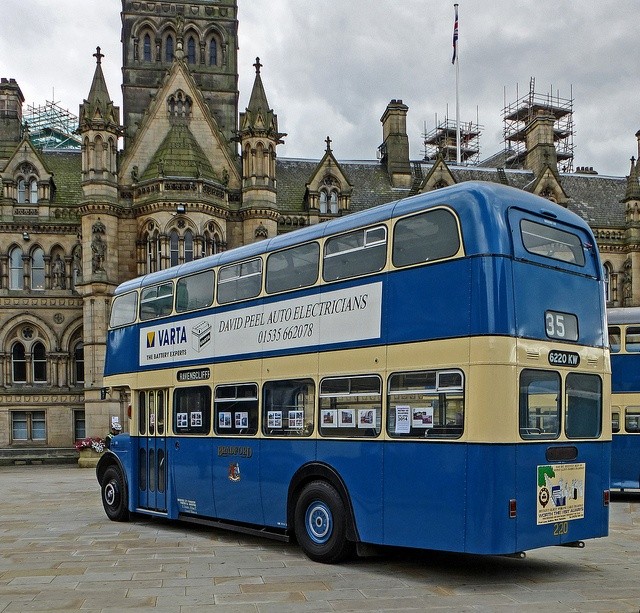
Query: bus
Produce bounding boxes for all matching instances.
[96,180,611,564]
[298,307,640,491]
